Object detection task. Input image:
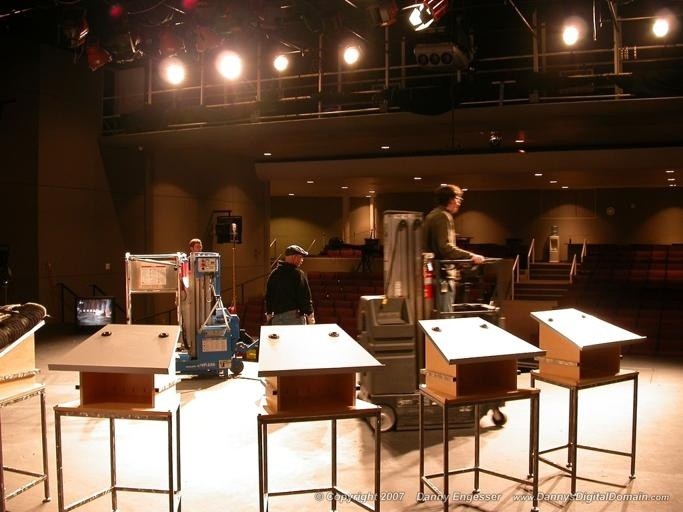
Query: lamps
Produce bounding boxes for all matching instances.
[85,33,113,71]
[406,0,448,32]
[515,128,526,143]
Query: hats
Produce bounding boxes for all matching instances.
[285,244,309,257]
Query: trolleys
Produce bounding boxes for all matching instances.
[175,255,273,380]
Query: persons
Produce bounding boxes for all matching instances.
[265,244,316,324]
[185,238,203,271]
[417,185,484,319]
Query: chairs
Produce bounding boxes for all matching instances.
[223,242,683,356]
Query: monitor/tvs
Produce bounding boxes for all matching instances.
[75,296,115,334]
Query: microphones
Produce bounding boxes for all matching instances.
[229,222,237,240]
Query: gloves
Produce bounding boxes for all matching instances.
[305,313,316,324]
[267,313,274,324]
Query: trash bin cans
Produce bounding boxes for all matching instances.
[549,236,559,263]
